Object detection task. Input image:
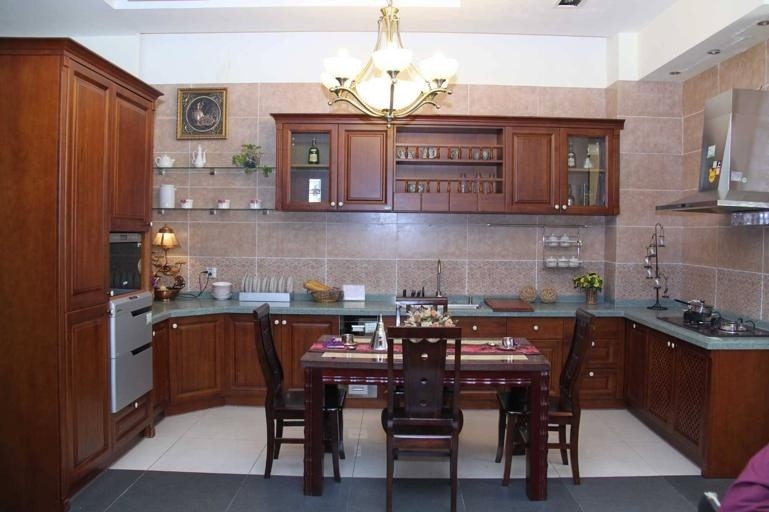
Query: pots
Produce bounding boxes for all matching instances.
[673,297,714,317]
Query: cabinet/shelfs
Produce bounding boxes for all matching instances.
[540,227,584,271]
[228,313,342,406]
[392,112,507,213]
[268,112,394,213]
[623,317,769,477]
[378,316,624,408]
[152,313,228,415]
[150,163,276,210]
[508,116,626,214]
[0,34,164,512]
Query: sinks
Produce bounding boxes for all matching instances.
[401,303,479,310]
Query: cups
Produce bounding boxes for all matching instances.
[250,199,262,209]
[180,200,194,208]
[450,149,489,160]
[502,336,513,349]
[341,334,354,345]
[218,200,230,207]
[213,282,232,297]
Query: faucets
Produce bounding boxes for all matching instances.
[435,260,443,298]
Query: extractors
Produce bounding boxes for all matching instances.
[654,87,769,215]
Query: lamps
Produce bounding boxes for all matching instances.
[326,0,454,119]
[152,223,186,301]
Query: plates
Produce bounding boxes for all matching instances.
[240,271,295,292]
[211,294,234,300]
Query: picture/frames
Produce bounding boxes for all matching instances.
[176,87,231,140]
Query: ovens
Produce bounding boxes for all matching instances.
[109,291,155,414]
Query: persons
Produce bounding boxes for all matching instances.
[716,444,769,512]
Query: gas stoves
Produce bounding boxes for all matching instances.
[654,310,769,339]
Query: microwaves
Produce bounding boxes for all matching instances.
[106,231,144,296]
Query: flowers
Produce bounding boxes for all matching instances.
[573,272,605,295]
[404,305,456,342]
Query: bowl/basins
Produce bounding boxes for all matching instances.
[309,288,341,303]
[405,184,425,192]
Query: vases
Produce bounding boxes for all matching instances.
[585,291,598,305]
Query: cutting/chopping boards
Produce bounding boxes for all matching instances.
[484,297,532,312]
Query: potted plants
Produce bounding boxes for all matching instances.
[232,142,274,178]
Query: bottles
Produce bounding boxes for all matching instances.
[460,174,496,193]
[159,183,175,208]
[370,313,388,351]
[310,138,320,164]
[584,150,593,169]
[546,255,579,267]
[567,184,576,206]
[548,233,571,246]
[567,143,576,168]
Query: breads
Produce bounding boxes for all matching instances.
[302,280,330,292]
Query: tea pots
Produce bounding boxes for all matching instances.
[397,148,415,159]
[190,143,208,168]
[154,154,176,168]
[420,147,438,158]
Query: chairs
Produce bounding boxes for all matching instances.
[381,326,465,512]
[252,302,346,483]
[495,308,596,485]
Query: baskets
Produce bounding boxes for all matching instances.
[312,290,341,302]
